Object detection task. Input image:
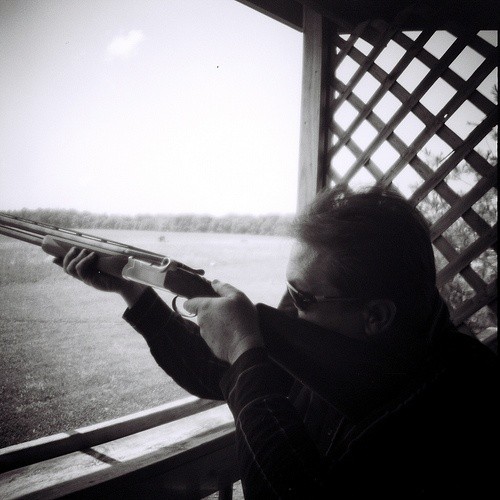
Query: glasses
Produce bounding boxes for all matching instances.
[285,282,370,310]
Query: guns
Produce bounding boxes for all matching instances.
[0,211,436,448]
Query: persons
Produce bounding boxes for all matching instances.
[51,185,500,500]
